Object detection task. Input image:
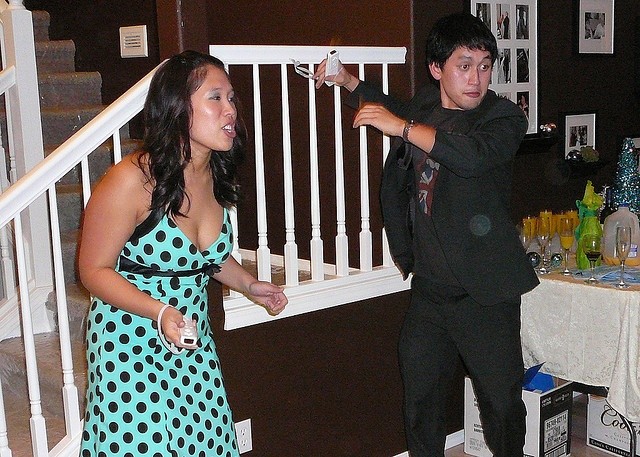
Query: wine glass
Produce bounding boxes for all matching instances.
[616,227,631,288]
[517,220,531,252]
[559,217,574,276]
[537,218,551,270]
[582,234,605,285]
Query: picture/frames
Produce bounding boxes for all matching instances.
[471,0,537,136]
[579,1,615,55]
[565,114,596,160]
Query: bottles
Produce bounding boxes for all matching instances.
[599,188,617,231]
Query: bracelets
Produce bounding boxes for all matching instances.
[157,305,185,355]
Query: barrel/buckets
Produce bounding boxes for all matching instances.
[603,203,640,268]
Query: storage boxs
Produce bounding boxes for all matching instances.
[586,394,640,457]
[463,375,571,456]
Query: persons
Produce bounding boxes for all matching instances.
[518,95,528,115]
[500,52,504,65]
[311,13,540,457]
[483,4,487,21]
[503,12,510,39]
[477,4,483,22]
[585,12,605,39]
[571,127,586,146]
[502,50,510,83]
[78,50,289,457]
[497,15,504,30]
[516,7,527,38]
[518,51,529,82]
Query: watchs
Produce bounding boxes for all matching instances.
[402,118,419,142]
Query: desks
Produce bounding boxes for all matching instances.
[521,234,640,421]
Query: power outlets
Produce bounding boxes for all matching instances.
[235,419,253,455]
